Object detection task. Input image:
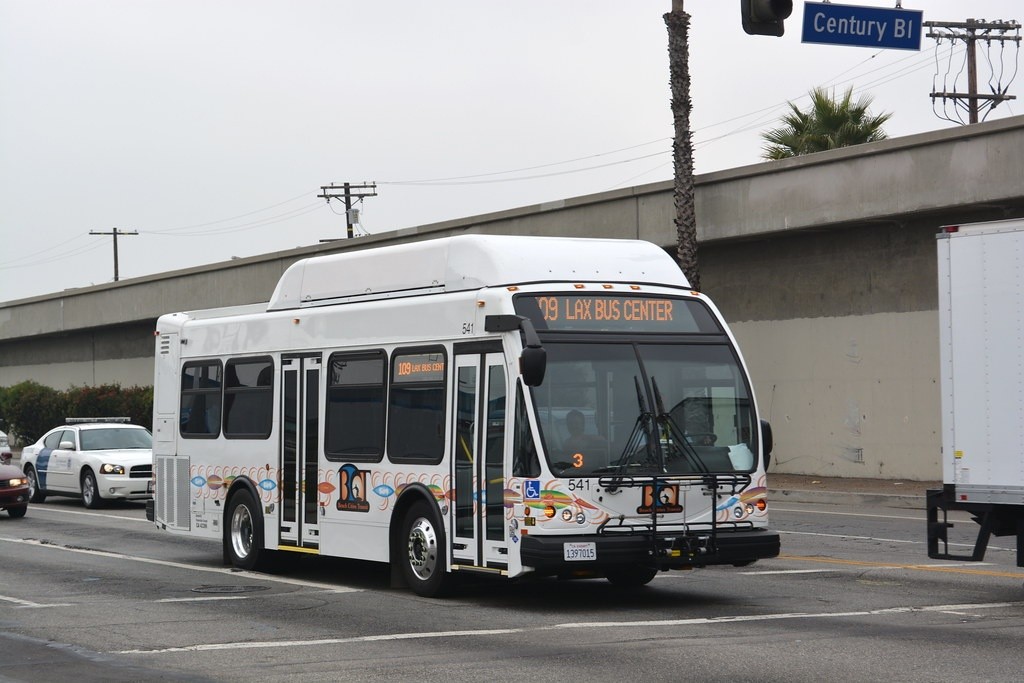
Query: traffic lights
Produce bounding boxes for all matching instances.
[741,0,793,37]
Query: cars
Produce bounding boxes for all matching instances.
[20,418,153,508]
[0,430,30,517]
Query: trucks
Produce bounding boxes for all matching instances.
[926,219,1024,567]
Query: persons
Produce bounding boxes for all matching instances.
[565,410,605,447]
[633,393,693,448]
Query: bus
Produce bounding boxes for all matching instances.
[146,234,780,599]
